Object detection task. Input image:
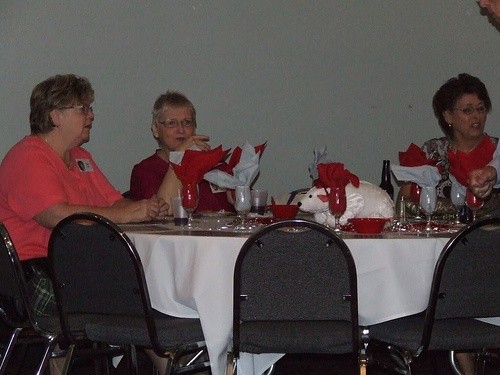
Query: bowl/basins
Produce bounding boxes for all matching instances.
[269,205,299,221]
[348,218,389,234]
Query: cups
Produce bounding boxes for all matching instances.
[250,189,268,216]
[170,188,189,227]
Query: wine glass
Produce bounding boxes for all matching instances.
[181,183,199,230]
[450,182,466,225]
[410,183,423,221]
[420,186,437,235]
[328,186,346,232]
[234,185,251,229]
[464,189,484,221]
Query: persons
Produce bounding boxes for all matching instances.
[0,72,168,375]
[130,91,236,211]
[466,142,499,198]
[395,72,499,221]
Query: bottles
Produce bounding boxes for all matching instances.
[397,195,408,224]
[379,159,394,202]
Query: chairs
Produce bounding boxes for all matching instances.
[47,212,211,375]
[225,218,368,375]
[0,221,123,375]
[368,217,500,375]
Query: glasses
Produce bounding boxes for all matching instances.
[59,104,92,115]
[454,106,488,115]
[160,118,194,127]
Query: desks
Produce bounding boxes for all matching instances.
[118,212,500,375]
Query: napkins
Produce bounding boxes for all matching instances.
[446,135,496,188]
[168,144,232,185]
[313,160,361,189]
[390,143,442,188]
[204,141,268,189]
